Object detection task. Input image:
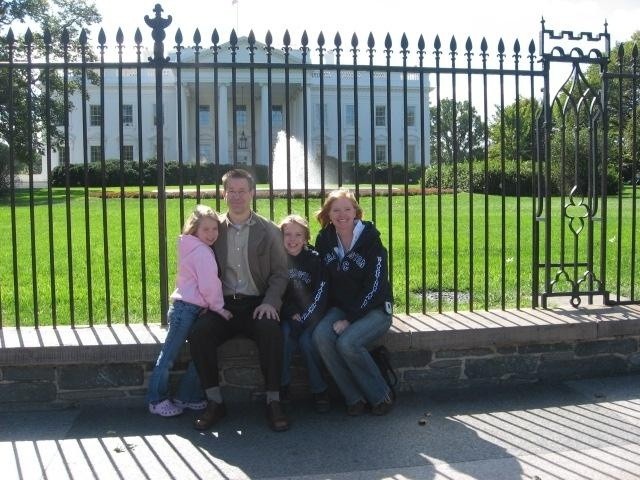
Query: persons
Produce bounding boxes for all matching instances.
[189,168,290,431]
[311,190,394,417]
[147,204,234,417]
[280,213,331,420]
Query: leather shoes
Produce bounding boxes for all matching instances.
[264,400,288,431]
[193,400,225,430]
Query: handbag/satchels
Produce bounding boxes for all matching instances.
[369,345,397,402]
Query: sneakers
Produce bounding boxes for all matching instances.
[173,398,208,410]
[347,401,365,416]
[278,384,291,405]
[313,387,330,413]
[148,398,183,417]
[371,391,393,416]
